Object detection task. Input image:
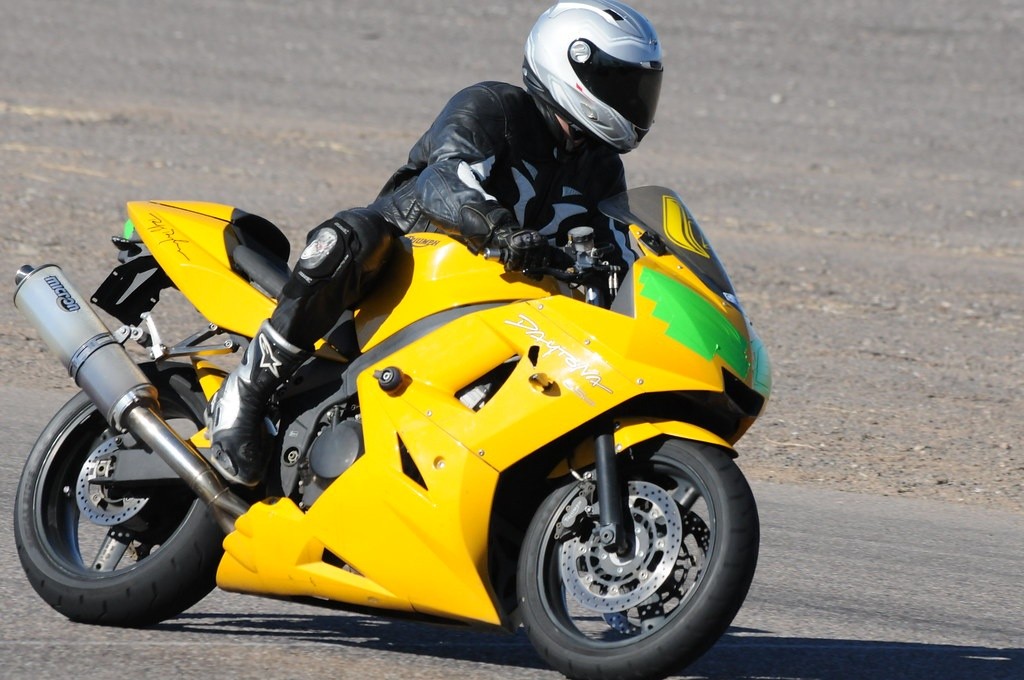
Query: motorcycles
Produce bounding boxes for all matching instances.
[13,186,772,680]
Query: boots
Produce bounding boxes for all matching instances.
[203,318,316,486]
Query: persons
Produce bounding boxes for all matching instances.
[204,0,663,485]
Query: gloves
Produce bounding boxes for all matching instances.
[458,198,548,281]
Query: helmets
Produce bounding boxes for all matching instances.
[522,0,664,154]
